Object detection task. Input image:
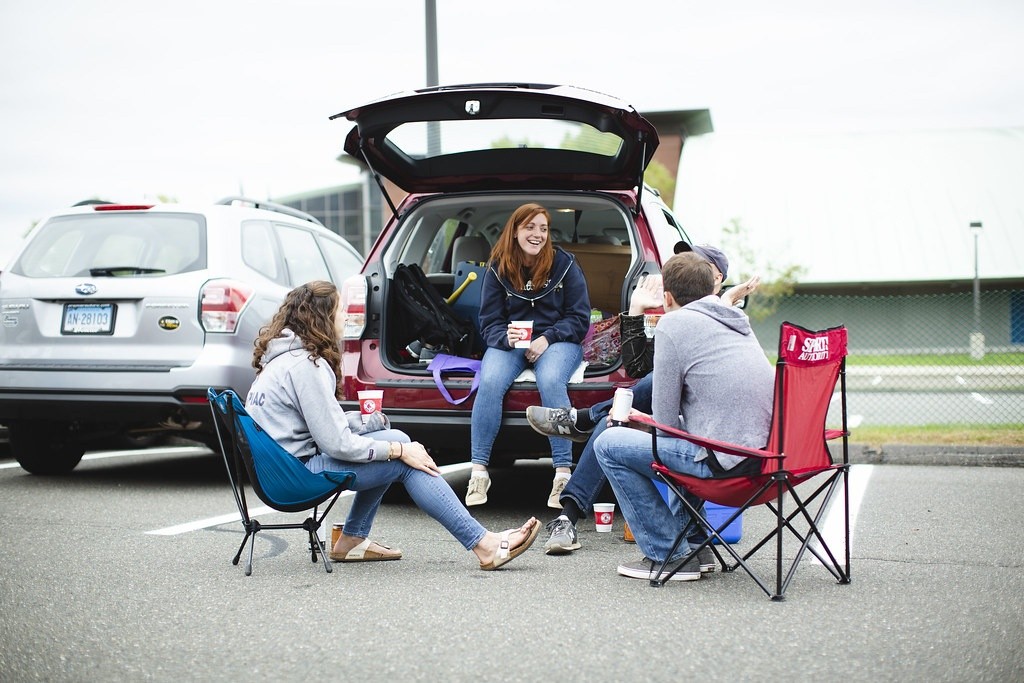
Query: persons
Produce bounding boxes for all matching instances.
[244,281,542,570]
[466,202,591,508]
[593,252,776,581]
[527,241,761,554]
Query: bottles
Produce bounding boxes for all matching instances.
[590,308,602,324]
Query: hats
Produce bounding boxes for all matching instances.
[673,240,729,283]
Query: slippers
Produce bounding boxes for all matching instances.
[330,537,402,562]
[479,519,543,570]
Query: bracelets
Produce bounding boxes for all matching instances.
[398,442,402,458]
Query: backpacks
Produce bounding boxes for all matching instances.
[393,262,475,354]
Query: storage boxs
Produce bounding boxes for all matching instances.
[652,479,745,545]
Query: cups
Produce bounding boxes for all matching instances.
[593,503,615,533]
[357,390,384,423]
[511,321,533,348]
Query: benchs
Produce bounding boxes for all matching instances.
[558,243,590,316]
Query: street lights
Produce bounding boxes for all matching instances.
[968,219,983,332]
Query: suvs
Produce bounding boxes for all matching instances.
[0,196,368,476]
[336,78,750,468]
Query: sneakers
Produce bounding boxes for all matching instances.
[547,474,571,509]
[617,550,702,581]
[694,546,716,573]
[527,406,596,443]
[465,476,493,506]
[405,338,424,359]
[419,343,449,366]
[544,518,584,555]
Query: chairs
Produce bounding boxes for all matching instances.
[631,320,855,599]
[206,385,357,576]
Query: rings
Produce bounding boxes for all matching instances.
[532,356,535,358]
[747,286,749,289]
